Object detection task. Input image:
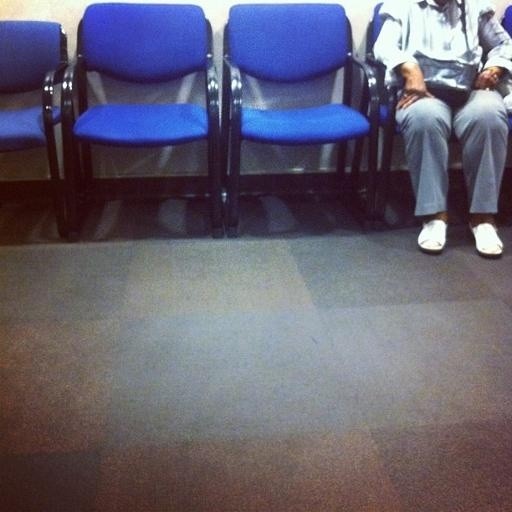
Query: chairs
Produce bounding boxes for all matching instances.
[0,22,83,235]
[502,6,512,132]
[221,4,380,239]
[61,4,224,243]
[350,13,457,231]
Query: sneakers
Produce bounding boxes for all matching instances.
[468,221,504,256]
[417,218,448,249]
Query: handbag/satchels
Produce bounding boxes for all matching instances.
[412,55,480,104]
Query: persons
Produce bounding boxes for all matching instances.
[497,5,511,117]
[374,1,512,255]
